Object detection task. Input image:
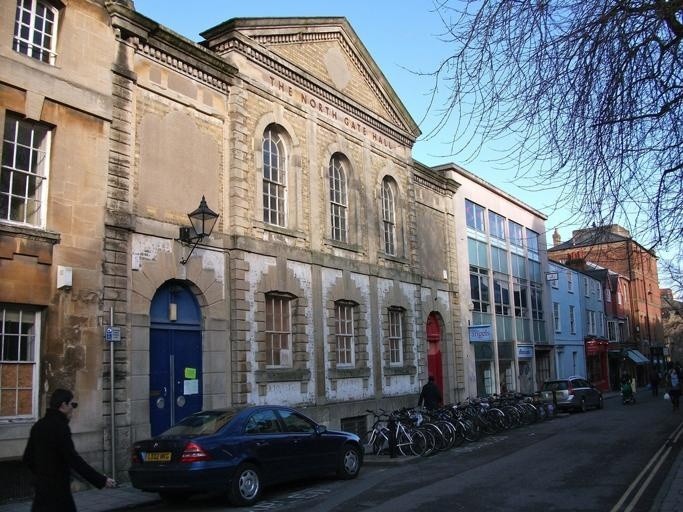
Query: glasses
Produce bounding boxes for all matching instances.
[68,402,78,409]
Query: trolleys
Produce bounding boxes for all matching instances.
[623,392,636,406]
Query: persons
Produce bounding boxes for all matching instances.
[20,387,119,511]
[618,359,682,413]
[417,375,444,411]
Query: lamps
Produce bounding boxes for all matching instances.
[179,195,220,264]
[632,325,640,335]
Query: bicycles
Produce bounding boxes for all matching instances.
[363,391,554,458]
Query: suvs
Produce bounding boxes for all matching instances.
[540,376,603,412]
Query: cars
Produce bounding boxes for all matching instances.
[128,405,364,507]
